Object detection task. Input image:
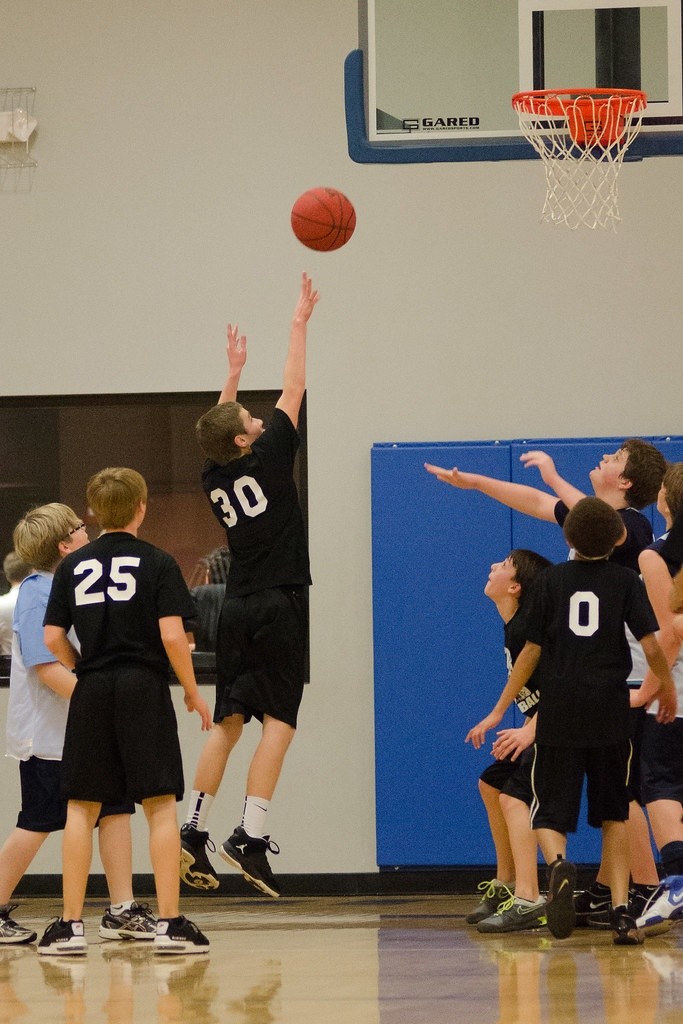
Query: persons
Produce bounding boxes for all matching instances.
[423,439,683,945]
[180,274,319,898]
[0,467,212,955]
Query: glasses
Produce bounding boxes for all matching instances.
[62,518,88,540]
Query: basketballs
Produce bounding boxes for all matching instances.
[291,188,357,251]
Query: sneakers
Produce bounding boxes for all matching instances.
[218,825,280,898]
[0,902,37,944]
[152,914,211,953]
[466,879,516,927]
[179,822,220,890]
[37,916,89,957]
[636,876,683,936]
[609,905,645,944]
[477,895,548,934]
[574,883,612,915]
[99,901,158,940]
[545,854,577,939]
[586,889,656,930]
